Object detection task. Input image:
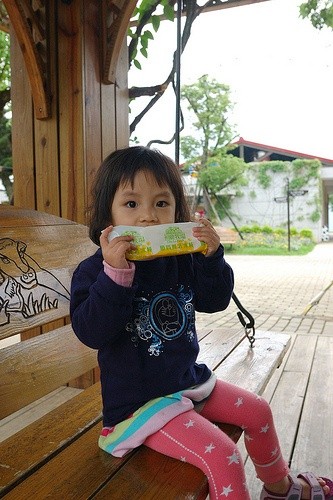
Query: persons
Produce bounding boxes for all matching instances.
[68,145,333,499]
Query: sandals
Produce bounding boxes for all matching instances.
[259,471,333,500]
